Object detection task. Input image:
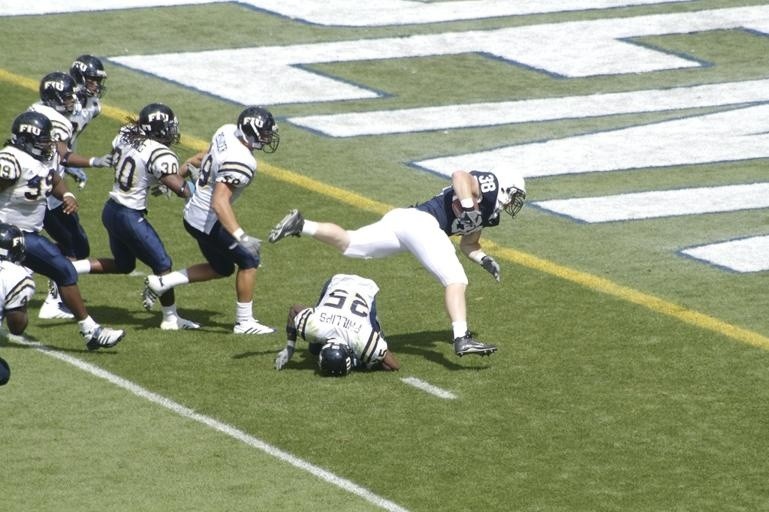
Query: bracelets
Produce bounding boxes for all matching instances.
[286,340,295,348]
[469,249,486,262]
[232,227,245,241]
[461,198,474,208]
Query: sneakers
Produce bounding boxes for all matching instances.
[79,325,124,352]
[232,319,274,335]
[142,275,166,310]
[269,209,304,243]
[454,333,498,356]
[39,302,74,318]
[47,279,59,299]
[160,318,201,330]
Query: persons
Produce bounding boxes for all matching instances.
[48,103,199,330]
[1,54,124,351]
[268,167,526,355]
[273,274,399,377]
[143,107,280,334]
[0,224,36,383]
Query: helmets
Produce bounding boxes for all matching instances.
[70,54,107,100]
[139,104,180,146]
[0,223,27,262]
[10,112,56,162]
[40,72,76,115]
[237,107,280,153]
[497,172,526,217]
[318,341,355,376]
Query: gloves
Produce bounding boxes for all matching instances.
[151,183,173,199]
[237,233,262,261]
[482,255,501,282]
[64,167,87,190]
[459,206,480,231]
[92,155,112,169]
[273,347,293,371]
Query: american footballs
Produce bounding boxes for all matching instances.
[451,199,480,219]
